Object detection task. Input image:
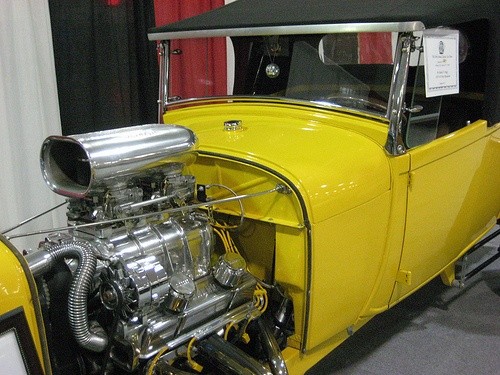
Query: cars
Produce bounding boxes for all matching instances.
[0,0,500,375]
